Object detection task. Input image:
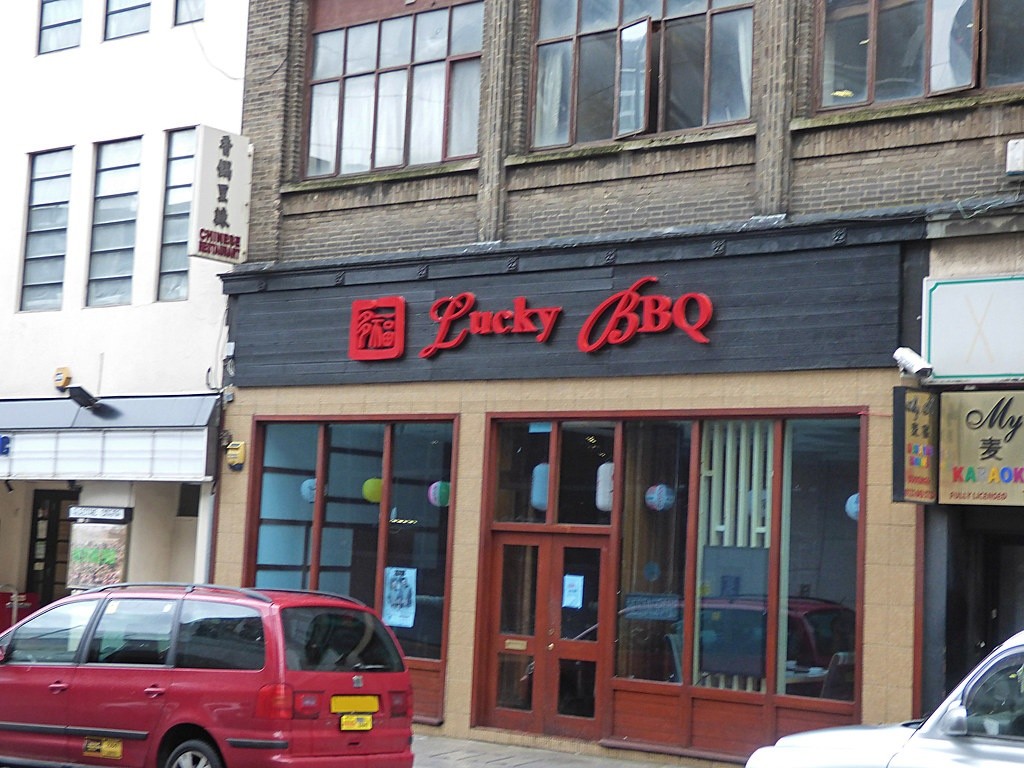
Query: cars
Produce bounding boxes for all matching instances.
[1,581,414,768]
[741,630,1024,768]
[517,593,857,718]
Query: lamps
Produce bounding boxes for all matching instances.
[66,387,97,407]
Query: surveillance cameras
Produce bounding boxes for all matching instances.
[893,347,933,379]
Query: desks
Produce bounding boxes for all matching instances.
[785,669,827,684]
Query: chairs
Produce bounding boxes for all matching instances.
[104,623,158,663]
[820,652,855,701]
[664,634,683,683]
